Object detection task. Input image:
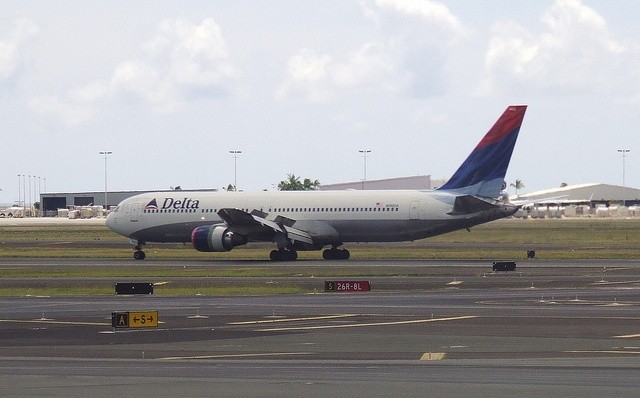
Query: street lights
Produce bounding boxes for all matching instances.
[229,151,241,192]
[100,152,112,192]
[617,150,631,187]
[358,150,371,181]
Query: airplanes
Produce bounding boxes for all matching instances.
[105,105,528,260]
